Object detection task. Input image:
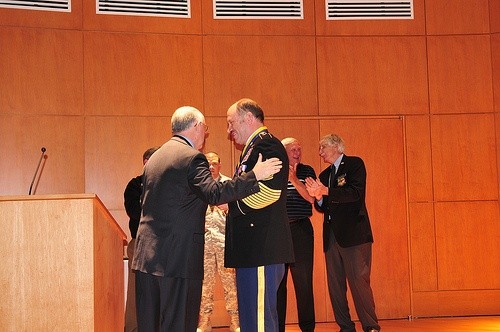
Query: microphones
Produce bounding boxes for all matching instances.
[29,147,46,195]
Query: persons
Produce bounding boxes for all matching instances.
[123,147,160,332]
[305,135,381,332]
[196,152,240,332]
[217,98,296,332]
[132,106,282,332]
[276,137,317,332]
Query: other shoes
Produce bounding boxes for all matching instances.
[229,314,240,332]
[367,327,380,332]
[197,315,212,332]
[340,328,356,332]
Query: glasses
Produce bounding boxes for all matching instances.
[194,120,208,132]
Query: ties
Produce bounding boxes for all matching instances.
[329,164,335,188]
